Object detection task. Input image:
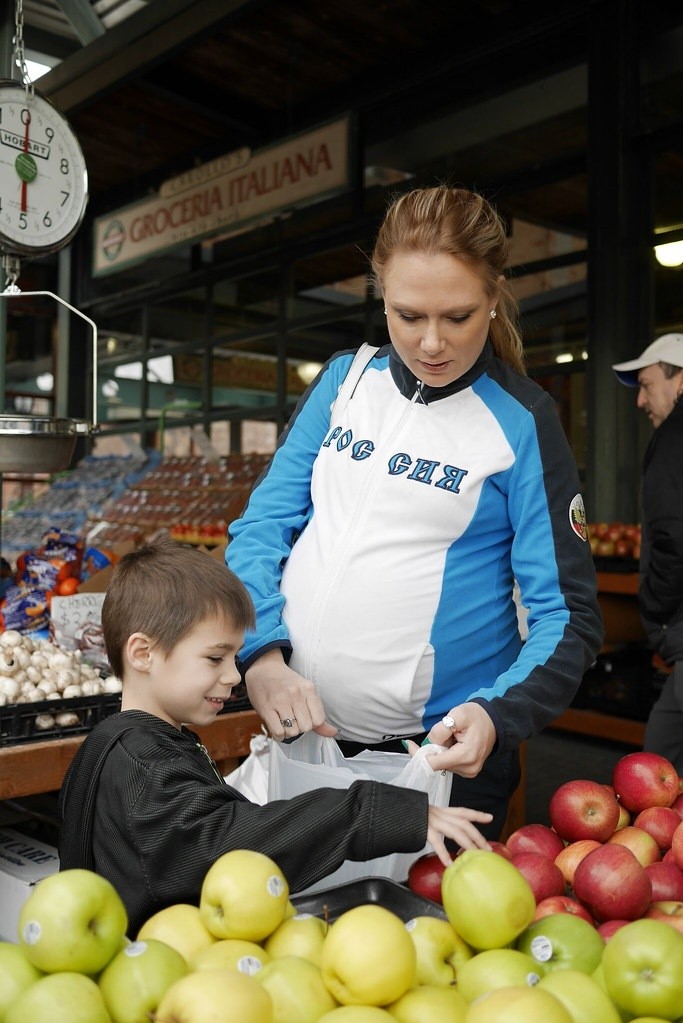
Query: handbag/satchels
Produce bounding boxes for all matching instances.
[268,731,453,898]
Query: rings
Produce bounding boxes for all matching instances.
[282,717,297,729]
[442,715,457,732]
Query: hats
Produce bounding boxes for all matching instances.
[611,332,683,389]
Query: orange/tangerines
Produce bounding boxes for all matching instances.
[45,558,81,609]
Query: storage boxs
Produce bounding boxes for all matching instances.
[51,564,110,665]
[0,688,123,751]
[0,830,60,945]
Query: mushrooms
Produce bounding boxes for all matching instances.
[0,629,123,729]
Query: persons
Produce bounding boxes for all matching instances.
[57,543,494,943]
[223,186,606,852]
[611,333,683,776]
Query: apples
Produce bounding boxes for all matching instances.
[0,847,683,1023]
[407,749,683,947]
[588,521,642,558]
